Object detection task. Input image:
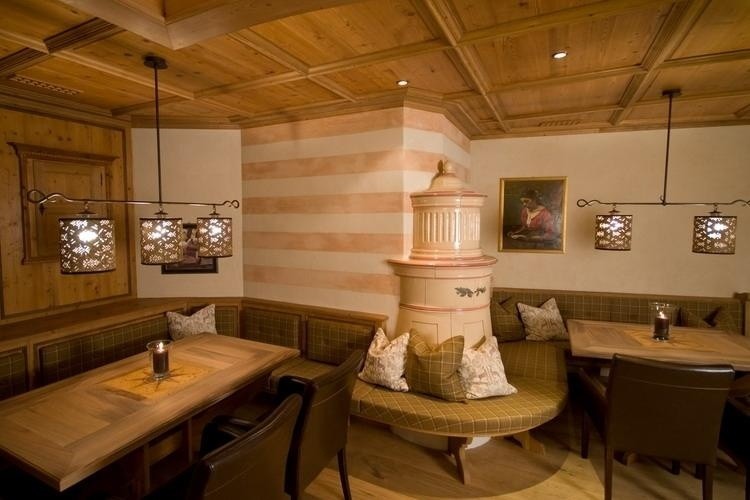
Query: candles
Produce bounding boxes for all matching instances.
[654,312,669,338]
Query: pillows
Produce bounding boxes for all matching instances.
[679,305,738,332]
[165,303,218,341]
[357,296,569,404]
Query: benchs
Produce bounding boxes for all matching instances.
[491,287,742,381]
[0,297,388,415]
[361,372,568,485]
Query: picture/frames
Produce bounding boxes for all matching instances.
[497,176,568,254]
[160,223,218,274]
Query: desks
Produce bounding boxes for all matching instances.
[0,332,300,500]
[567,317,750,465]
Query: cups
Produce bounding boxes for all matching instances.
[147,339,174,380]
[649,302,676,341]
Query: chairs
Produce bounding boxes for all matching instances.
[577,353,735,500]
[110,349,366,500]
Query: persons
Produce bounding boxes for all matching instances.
[506,188,561,242]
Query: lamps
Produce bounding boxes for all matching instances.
[577,89,750,255]
[27,56,240,275]
